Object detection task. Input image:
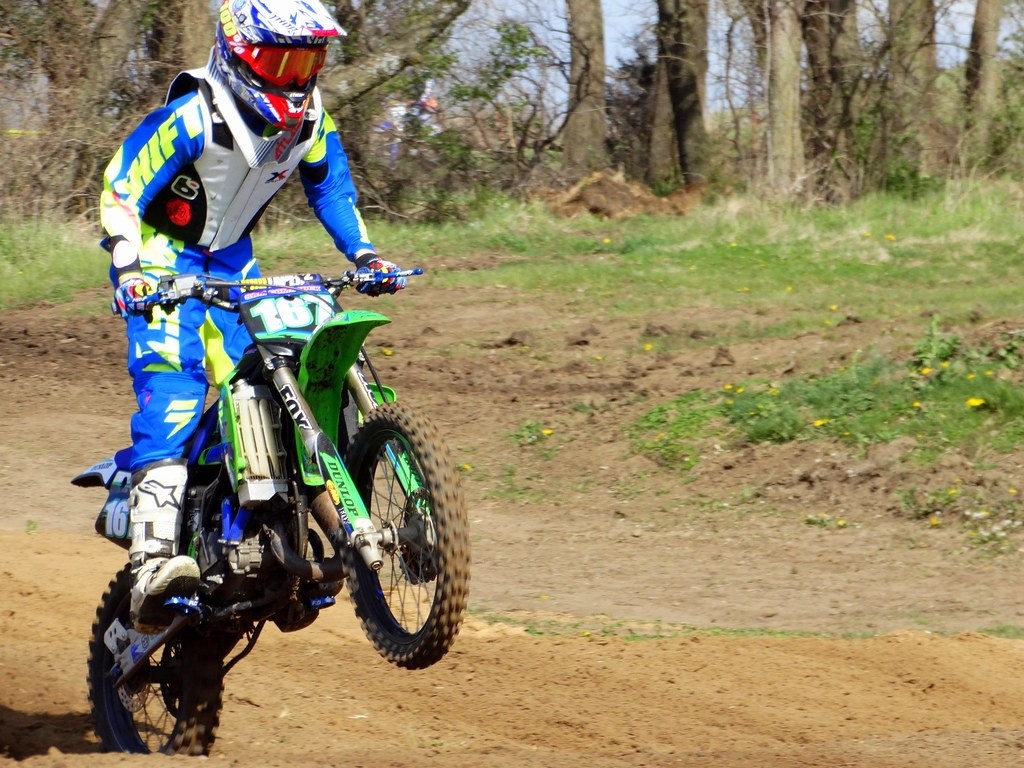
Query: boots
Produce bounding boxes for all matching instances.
[127,458,201,635]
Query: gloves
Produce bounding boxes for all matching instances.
[110,272,154,324]
[354,253,408,297]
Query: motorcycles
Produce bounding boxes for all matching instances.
[70,266,474,758]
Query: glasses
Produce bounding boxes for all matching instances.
[220,6,328,85]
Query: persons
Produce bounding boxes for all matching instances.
[100,0,407,634]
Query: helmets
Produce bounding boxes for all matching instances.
[205,0,347,130]
